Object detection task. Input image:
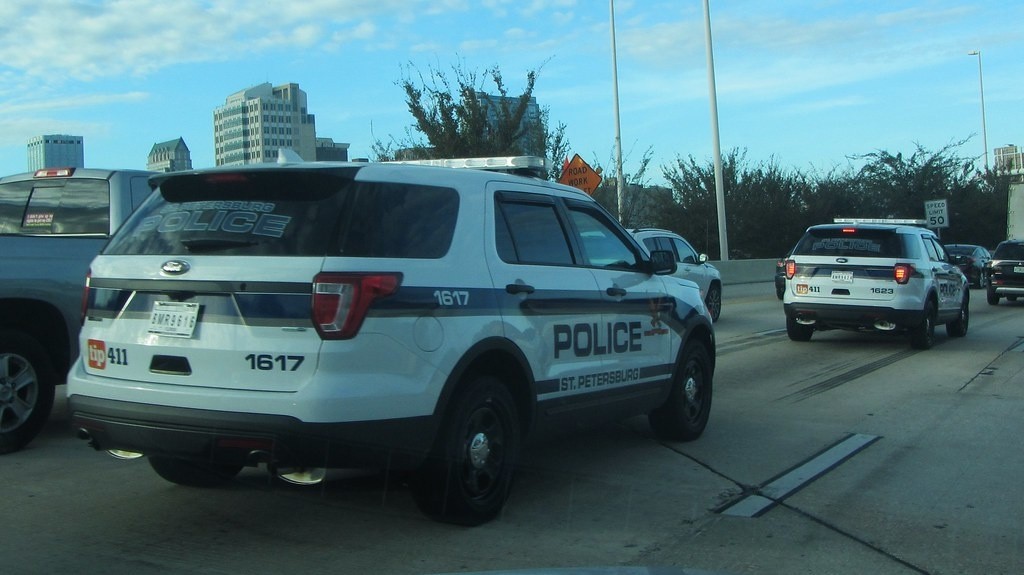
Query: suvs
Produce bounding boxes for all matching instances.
[579,227,722,323]
[64,148,717,532]
[0,169,180,458]
[783,218,970,350]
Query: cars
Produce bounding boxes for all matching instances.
[986,240,1024,305]
[944,243,991,287]
[775,249,794,298]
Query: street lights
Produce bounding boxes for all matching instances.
[968,50,988,169]
